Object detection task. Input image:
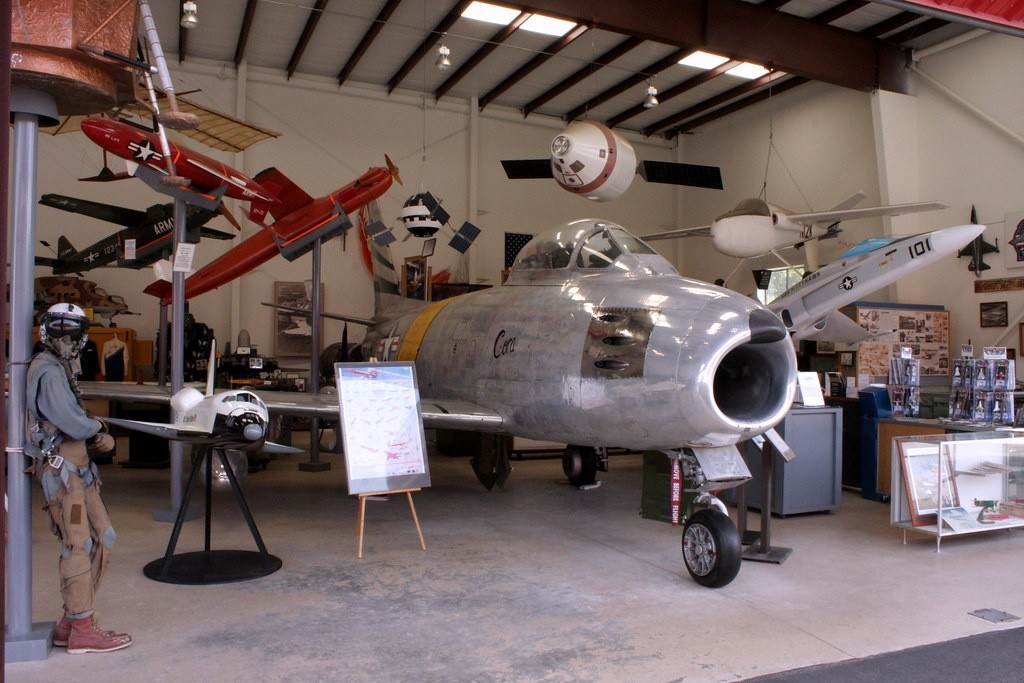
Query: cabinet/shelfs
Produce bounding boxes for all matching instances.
[639,390,1024,554]
[30,326,135,435]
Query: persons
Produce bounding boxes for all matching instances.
[24,302,132,656]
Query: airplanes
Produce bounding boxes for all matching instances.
[2,117,988,589]
[958,203,1003,281]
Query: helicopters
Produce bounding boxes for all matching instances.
[4,238,142,331]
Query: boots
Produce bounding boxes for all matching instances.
[53,604,115,646]
[66,610,133,654]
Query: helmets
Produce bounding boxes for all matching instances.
[38,303,89,357]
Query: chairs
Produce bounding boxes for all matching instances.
[552,248,583,269]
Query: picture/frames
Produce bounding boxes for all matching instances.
[272,281,324,358]
[979,302,1008,328]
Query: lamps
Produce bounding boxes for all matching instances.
[643,78,658,109]
[179,0,197,30]
[436,31,451,70]
